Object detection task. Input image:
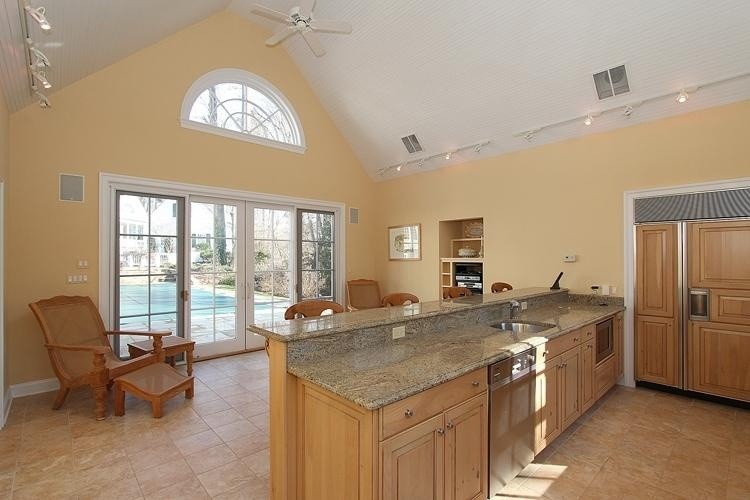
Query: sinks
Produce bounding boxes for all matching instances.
[489,320,556,334]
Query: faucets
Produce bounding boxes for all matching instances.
[510,300,520,309]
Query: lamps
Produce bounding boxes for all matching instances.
[513,84,698,141]
[374,140,491,178]
[17,0,55,110]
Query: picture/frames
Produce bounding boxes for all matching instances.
[387,222,422,262]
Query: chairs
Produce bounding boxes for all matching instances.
[264,301,345,358]
[346,278,512,312]
[28,295,174,423]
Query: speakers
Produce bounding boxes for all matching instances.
[59,173,84,203]
[349,207,359,224]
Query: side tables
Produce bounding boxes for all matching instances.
[126,334,196,379]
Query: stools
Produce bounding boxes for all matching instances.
[111,361,195,419]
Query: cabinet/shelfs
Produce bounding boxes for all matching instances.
[295,361,489,500]
[595,313,625,400]
[535,323,595,460]
[440,236,484,300]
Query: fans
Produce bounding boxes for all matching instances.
[247,1,354,58]
